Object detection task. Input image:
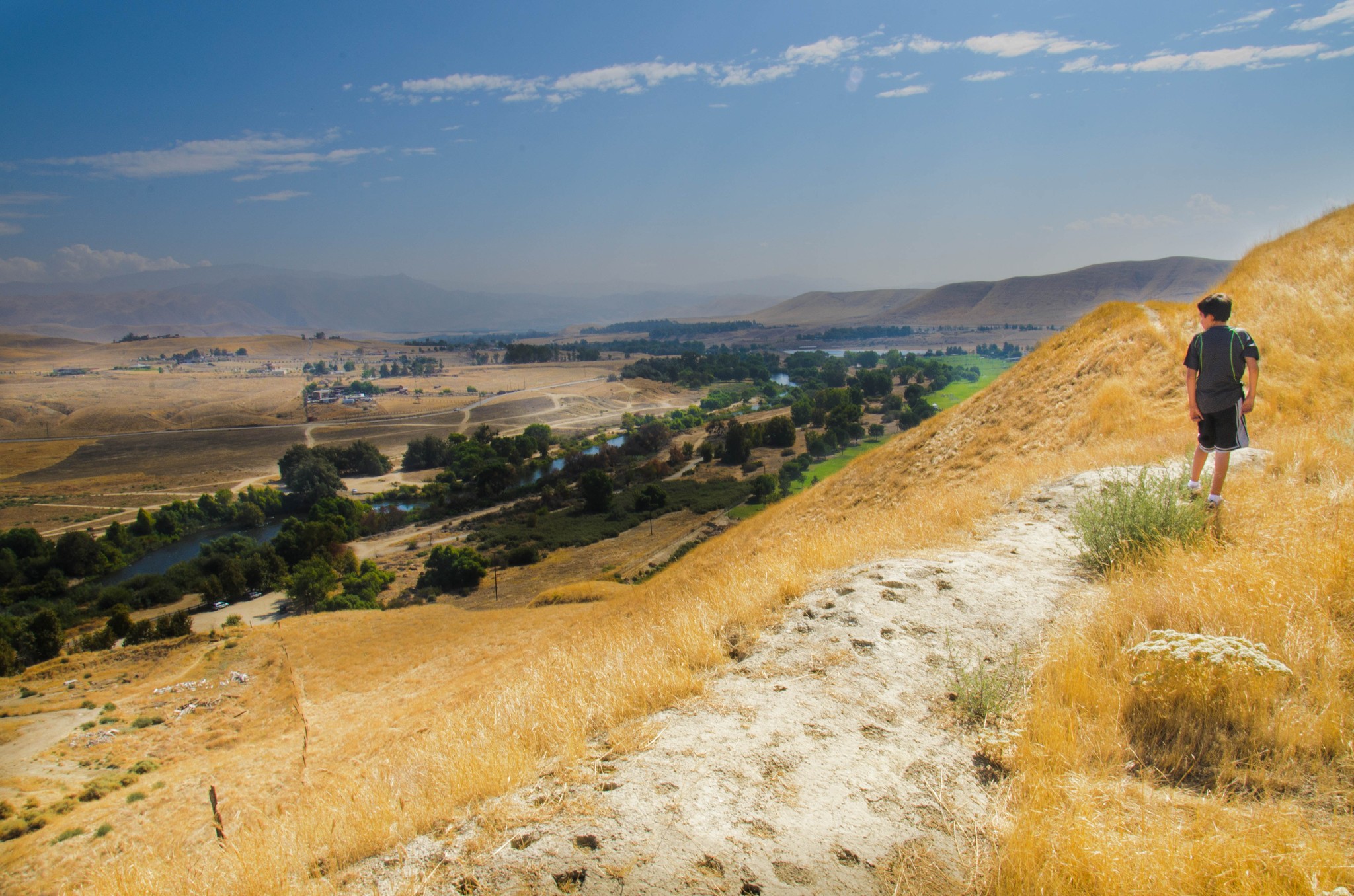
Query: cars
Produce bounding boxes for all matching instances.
[352,488,357,492]
[245,591,263,600]
[212,601,229,611]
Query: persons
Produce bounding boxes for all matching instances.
[1183,291,1260,509]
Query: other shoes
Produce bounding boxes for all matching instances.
[1204,497,1229,510]
[1185,484,1201,499]
